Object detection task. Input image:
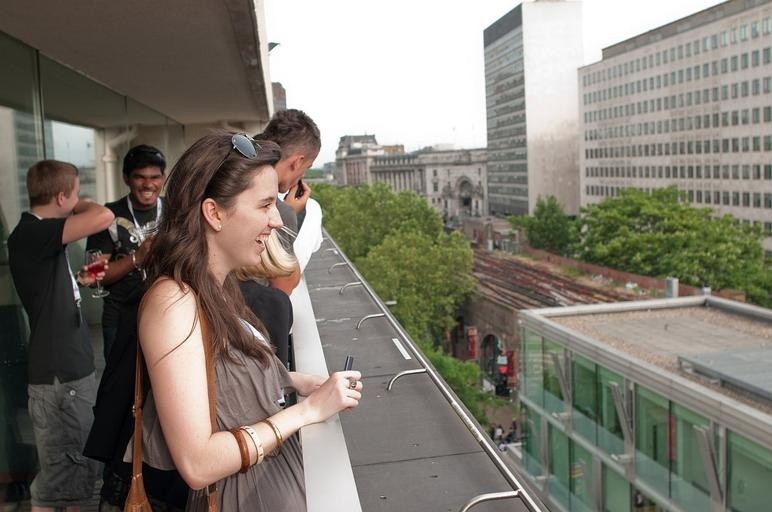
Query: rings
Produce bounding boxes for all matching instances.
[348,377,356,390]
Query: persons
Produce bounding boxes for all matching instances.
[509,427,516,439]
[635,489,644,512]
[136,128,364,512]
[279,195,325,272]
[87,144,163,504]
[497,424,504,441]
[8,160,115,512]
[272,108,321,238]
[113,207,302,511]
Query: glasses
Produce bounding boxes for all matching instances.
[124,149,161,157]
[207,133,263,189]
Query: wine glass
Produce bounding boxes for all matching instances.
[84,248,110,299]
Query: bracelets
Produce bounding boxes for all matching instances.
[232,418,284,473]
